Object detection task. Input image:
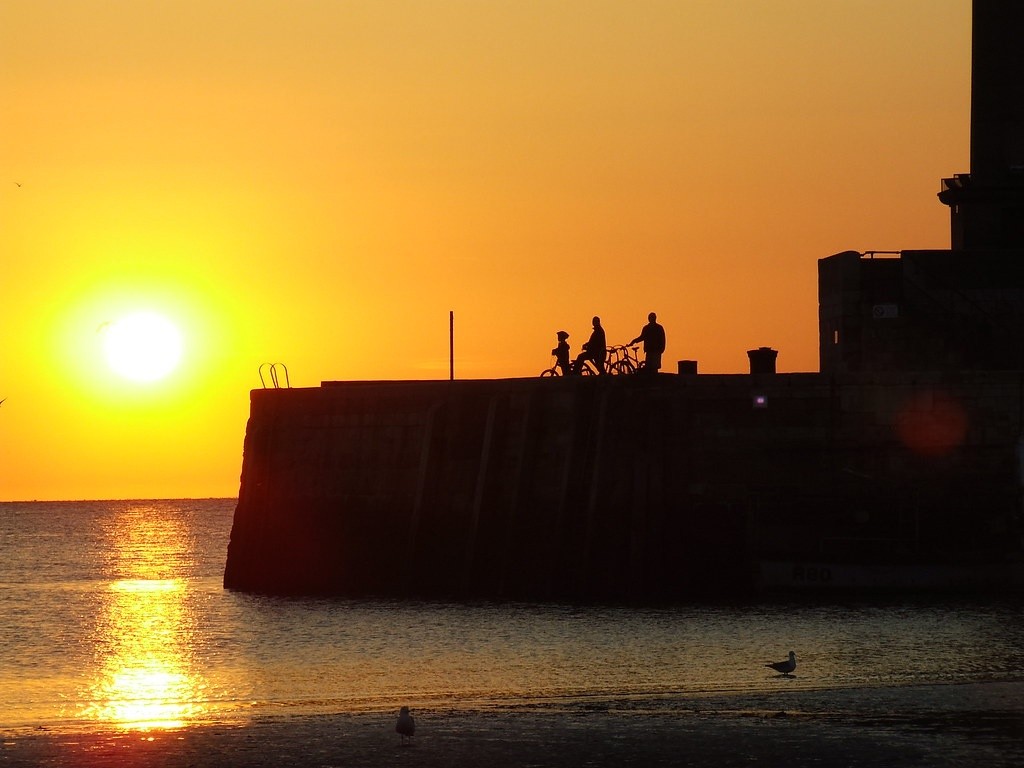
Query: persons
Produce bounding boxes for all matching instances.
[551,331,571,376]
[629,313,666,374]
[572,316,606,375]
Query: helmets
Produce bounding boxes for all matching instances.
[557,330,569,339]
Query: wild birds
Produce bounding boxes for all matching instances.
[765,651,797,677]
[395,705,416,747]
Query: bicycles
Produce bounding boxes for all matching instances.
[540,342,648,376]
